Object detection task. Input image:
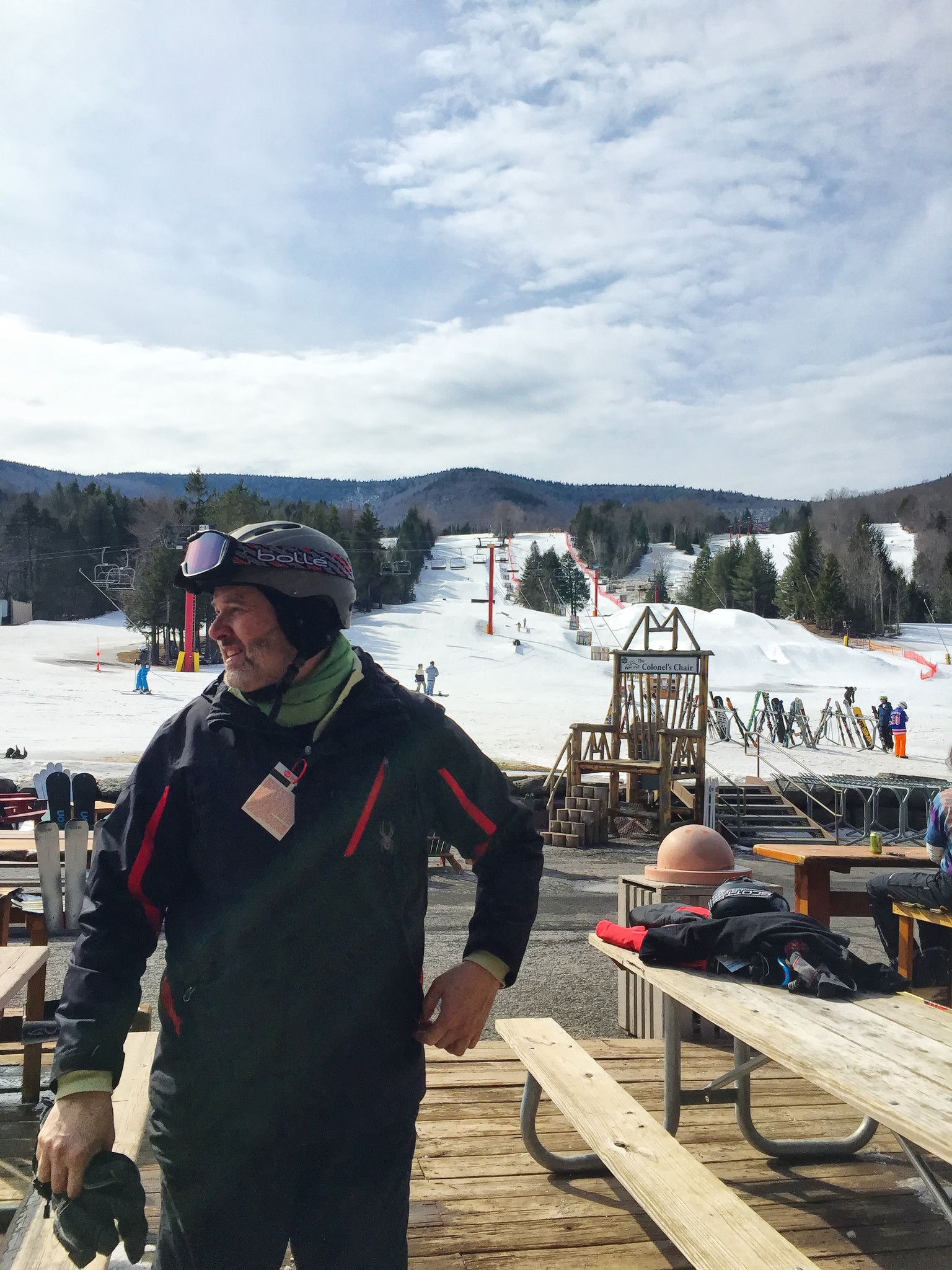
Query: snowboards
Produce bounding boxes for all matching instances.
[771,698,786,743]
[71,773,97,831]
[852,707,874,750]
[433,694,449,697]
[45,771,72,831]
[714,696,731,741]
[603,693,613,725]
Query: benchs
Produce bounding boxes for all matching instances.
[0,861,66,945]
[890,899,952,992]
[495,1017,821,1270]
[0,792,50,830]
[0,1006,160,1270]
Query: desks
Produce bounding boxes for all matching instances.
[588,932,952,1229]
[752,844,940,933]
[0,946,51,1101]
[69,800,116,827]
[0,829,94,861]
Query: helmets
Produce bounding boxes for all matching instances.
[898,701,907,709]
[707,875,790,920]
[853,687,857,691]
[430,661,435,665]
[225,519,356,648]
[882,695,888,702]
[138,645,149,651]
[418,663,423,667]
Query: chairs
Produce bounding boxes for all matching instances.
[426,828,464,876]
[567,606,715,847]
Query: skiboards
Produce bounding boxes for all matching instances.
[32,761,77,809]
[682,690,721,740]
[725,697,758,748]
[32,816,107,934]
[620,684,641,732]
[771,697,821,751]
[813,697,866,749]
[744,690,778,743]
[643,692,664,723]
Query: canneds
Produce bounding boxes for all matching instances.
[870,832,882,854]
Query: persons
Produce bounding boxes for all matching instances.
[516,622,521,632]
[35,520,546,1270]
[416,664,427,693]
[425,661,439,696]
[878,695,894,751]
[844,686,857,707]
[134,645,151,693]
[890,701,909,759]
[866,746,952,982]
[524,618,527,628]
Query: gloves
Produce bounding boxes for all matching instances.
[31,1094,149,1270]
[134,659,141,666]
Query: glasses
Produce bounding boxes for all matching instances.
[879,696,885,700]
[904,706,908,709]
[173,530,236,594]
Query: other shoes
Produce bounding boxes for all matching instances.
[895,755,900,757]
[134,688,143,691]
[888,947,952,988]
[886,748,889,751]
[140,689,149,692]
[899,755,909,759]
[889,748,893,752]
